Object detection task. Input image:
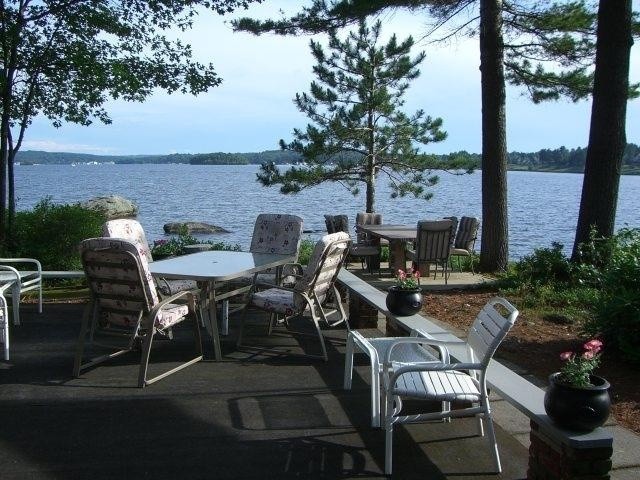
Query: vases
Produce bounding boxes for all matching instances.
[544,372,612,435]
[385,285,424,317]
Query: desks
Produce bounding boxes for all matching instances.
[147,250,296,363]
[370,229,418,276]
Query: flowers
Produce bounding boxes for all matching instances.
[557,339,604,387]
[395,266,423,291]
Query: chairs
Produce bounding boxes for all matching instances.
[381,295,519,477]
[73,235,205,389]
[354,213,390,275]
[323,214,382,277]
[214,213,303,331]
[100,218,199,342]
[0,258,44,327]
[441,216,458,272]
[0,295,14,360]
[434,216,480,279]
[235,230,352,363]
[406,219,454,288]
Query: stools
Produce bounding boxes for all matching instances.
[342,328,452,429]
